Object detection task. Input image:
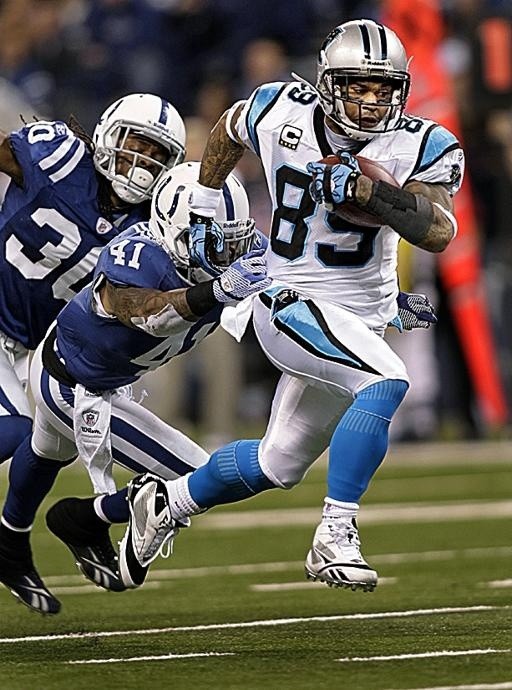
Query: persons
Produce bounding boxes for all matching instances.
[116,18,466,591]
[2,160,437,615]
[1,2,512,463]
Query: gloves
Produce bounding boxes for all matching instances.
[187,215,222,273]
[393,293,437,332]
[211,250,272,302]
[305,153,364,205]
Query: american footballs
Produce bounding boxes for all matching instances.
[318,156,399,228]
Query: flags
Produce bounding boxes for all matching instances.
[380,1,508,439]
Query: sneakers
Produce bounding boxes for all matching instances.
[2,562,61,615]
[305,523,378,593]
[46,497,126,591]
[118,474,189,588]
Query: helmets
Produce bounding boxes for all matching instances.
[92,92,187,204]
[150,162,255,286]
[318,19,409,140]
